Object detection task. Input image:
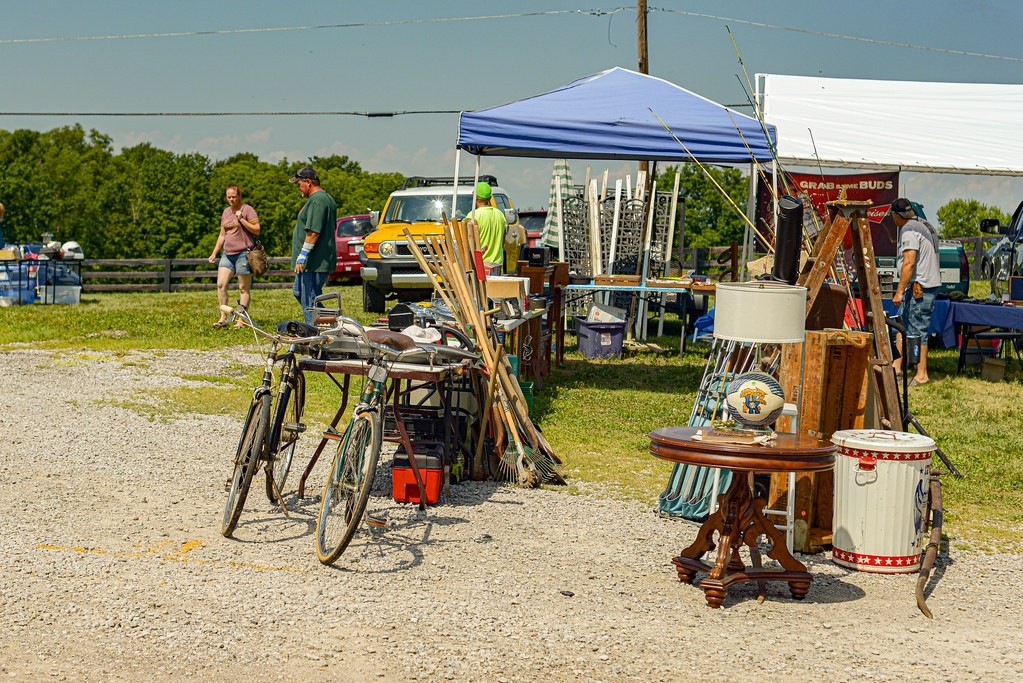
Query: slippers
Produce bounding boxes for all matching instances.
[907,377,931,386]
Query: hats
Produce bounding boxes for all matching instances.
[891,197,912,213]
[288,167,319,184]
[472,182,492,198]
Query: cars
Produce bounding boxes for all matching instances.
[979,200,1023,298]
[0,245,82,305]
[326,215,388,287]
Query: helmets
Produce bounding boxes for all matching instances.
[62,241,85,260]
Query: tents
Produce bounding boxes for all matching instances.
[744,72,1023,269]
[448,67,781,274]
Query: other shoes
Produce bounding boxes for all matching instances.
[213,322,228,328]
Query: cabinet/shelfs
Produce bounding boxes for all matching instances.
[372,260,571,385]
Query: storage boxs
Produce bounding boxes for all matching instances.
[595,274,640,286]
[0,289,35,304]
[765,329,873,555]
[0,250,18,260]
[520,382,534,417]
[41,285,82,304]
[392,441,445,504]
[958,346,997,366]
[573,316,627,359]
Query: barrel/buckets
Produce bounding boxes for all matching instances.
[959,346,997,374]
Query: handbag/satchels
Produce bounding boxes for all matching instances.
[247,245,269,277]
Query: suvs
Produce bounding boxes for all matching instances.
[514,208,548,247]
[358,176,519,314]
[938,239,969,298]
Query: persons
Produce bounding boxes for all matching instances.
[463,183,509,324]
[889,199,943,390]
[288,167,336,344]
[0,203,17,250]
[205,184,260,329]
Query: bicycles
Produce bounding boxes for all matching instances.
[316,316,438,565]
[210,299,335,538]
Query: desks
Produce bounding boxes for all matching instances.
[649,427,839,608]
[0,259,85,305]
[883,297,1023,378]
[543,283,688,361]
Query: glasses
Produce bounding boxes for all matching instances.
[295,173,301,178]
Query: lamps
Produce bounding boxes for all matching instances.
[713,282,807,432]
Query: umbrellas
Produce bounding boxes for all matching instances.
[539,159,584,263]
